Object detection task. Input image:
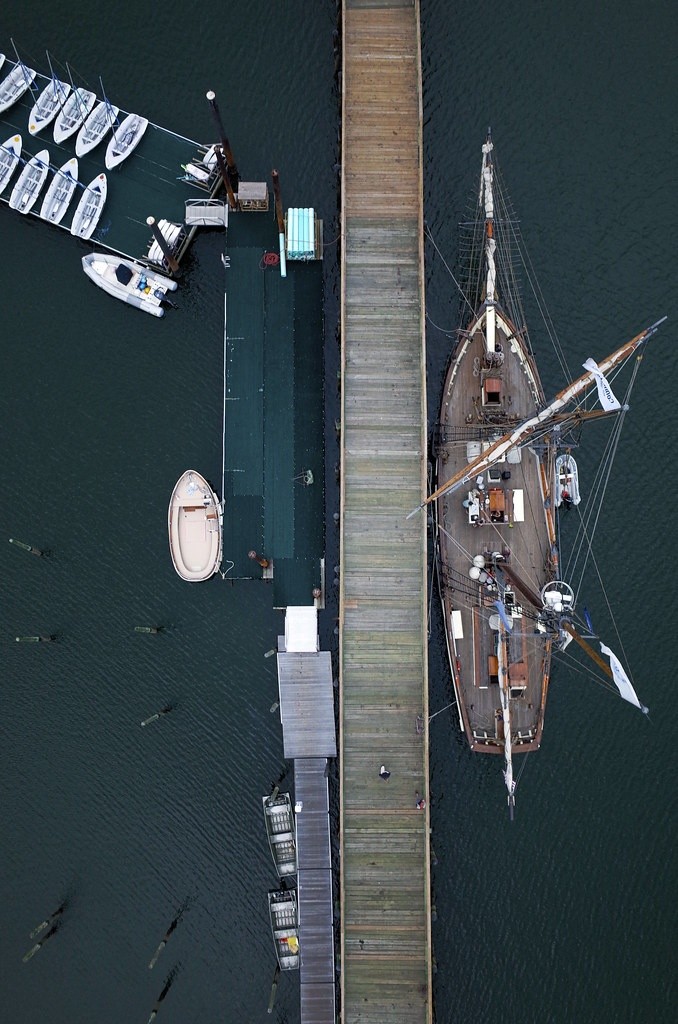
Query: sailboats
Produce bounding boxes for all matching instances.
[405,125,670,820]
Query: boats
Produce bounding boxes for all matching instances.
[65,62,120,158]
[22,149,78,225]
[0,134,23,195]
[11,37,71,137]
[45,49,96,145]
[267,888,300,972]
[49,162,107,240]
[0,144,50,215]
[0,64,36,113]
[98,75,149,170]
[167,469,224,582]
[263,792,297,879]
[81,252,179,317]
